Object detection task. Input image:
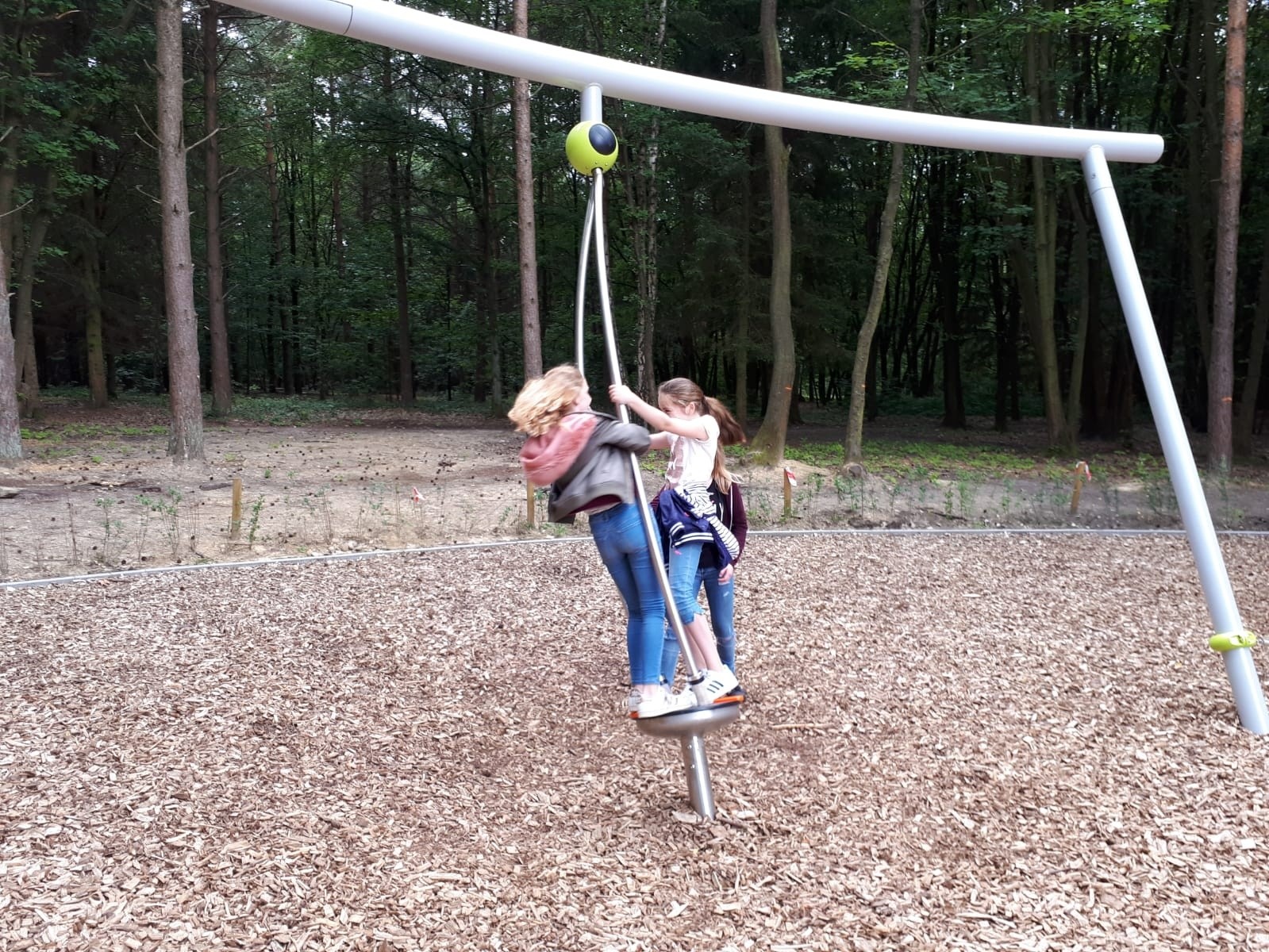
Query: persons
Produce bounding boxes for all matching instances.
[609,378,737,701]
[507,366,692,718]
[661,443,746,702]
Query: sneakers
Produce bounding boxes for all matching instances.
[683,665,738,706]
[628,677,692,718]
